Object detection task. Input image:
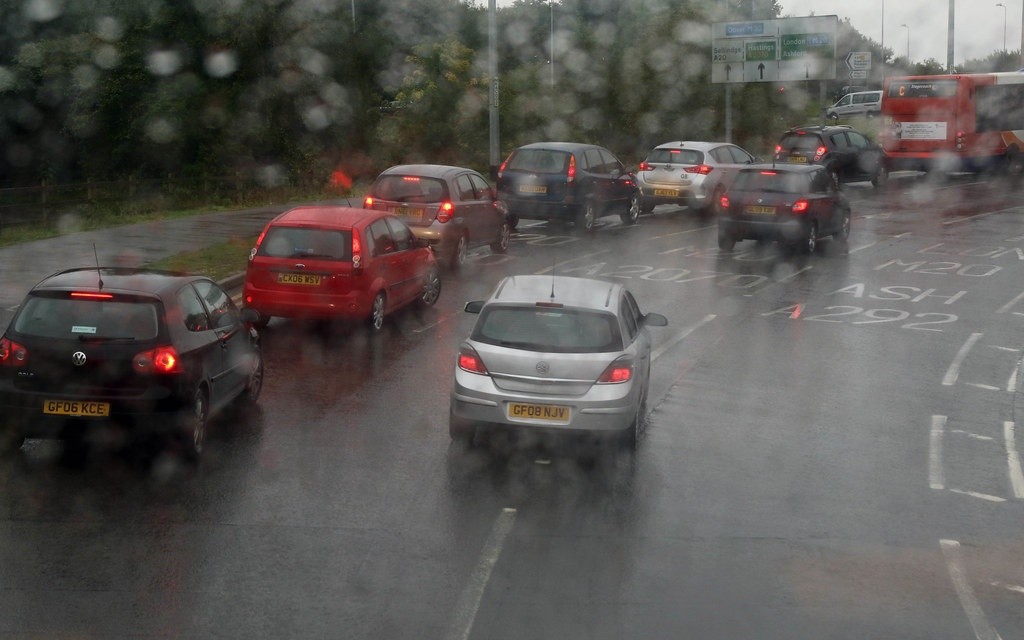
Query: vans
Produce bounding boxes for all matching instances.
[825,90,884,121]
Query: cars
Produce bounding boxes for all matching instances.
[496,142,643,237]
[1,264,265,472]
[832,86,870,104]
[634,141,765,222]
[241,205,444,337]
[447,274,670,451]
[363,164,514,274]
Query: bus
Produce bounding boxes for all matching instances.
[871,69,1024,182]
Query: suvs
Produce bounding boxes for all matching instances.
[774,123,890,191]
[717,162,852,257]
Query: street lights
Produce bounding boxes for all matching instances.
[902,24,910,63]
[995,2,1008,54]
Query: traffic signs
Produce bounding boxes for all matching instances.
[709,15,839,84]
[849,71,870,81]
[846,53,871,69]
